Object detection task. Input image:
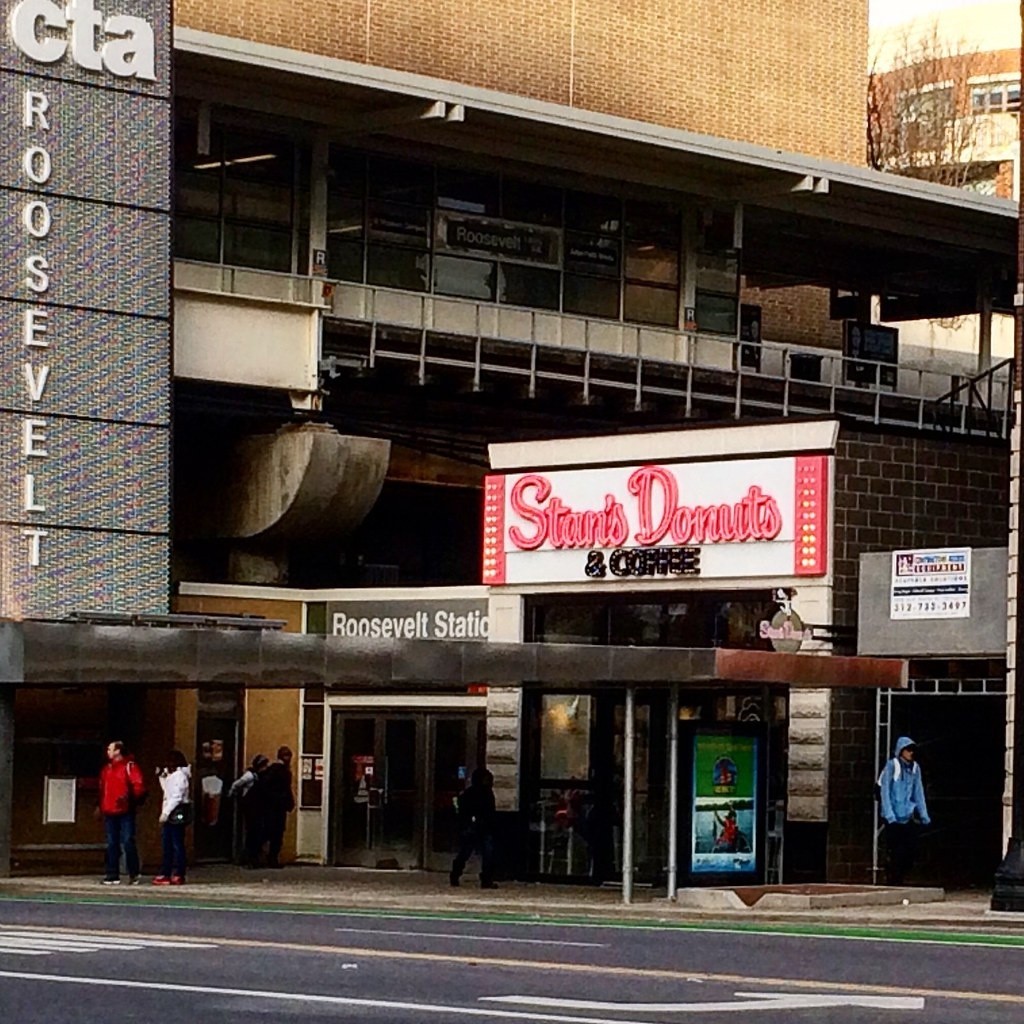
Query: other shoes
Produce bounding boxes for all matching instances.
[450,871,460,886]
[151,874,186,885]
[481,882,499,889]
[128,874,142,885]
[101,877,121,885]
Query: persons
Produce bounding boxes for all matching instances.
[91,740,149,885]
[712,802,739,853]
[228,746,296,869]
[151,750,195,885]
[442,769,499,890]
[875,736,932,885]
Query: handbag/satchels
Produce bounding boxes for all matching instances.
[168,803,196,827]
